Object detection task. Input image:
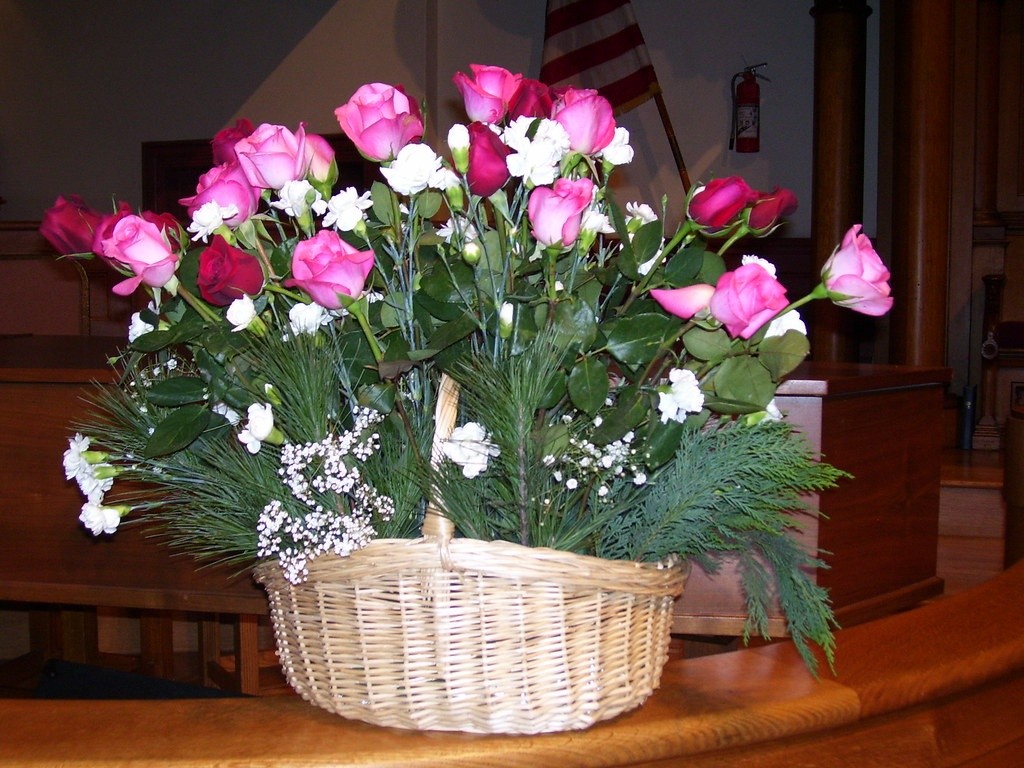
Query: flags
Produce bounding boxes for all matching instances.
[536,2,663,121]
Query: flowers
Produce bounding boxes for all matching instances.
[38,64,896,683]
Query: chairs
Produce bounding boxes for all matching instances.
[0,217,291,692]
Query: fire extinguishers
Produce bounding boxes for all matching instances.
[729,62,772,153]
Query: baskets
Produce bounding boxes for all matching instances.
[251,372,692,733]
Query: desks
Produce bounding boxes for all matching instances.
[1,334,282,692]
